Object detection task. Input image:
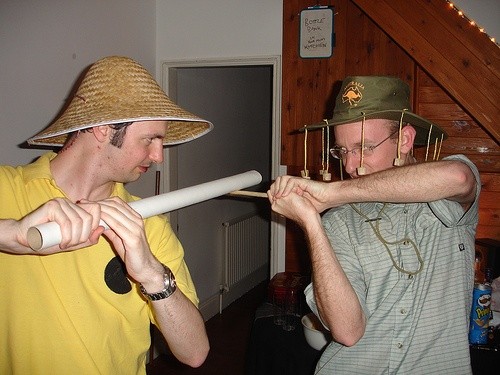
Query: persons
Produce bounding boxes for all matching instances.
[268,76,481,375]
[0,56,210,375]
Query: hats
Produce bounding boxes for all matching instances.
[299,76,448,148]
[27,55,214,146]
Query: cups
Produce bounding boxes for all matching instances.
[469,284,492,344]
[273,287,306,332]
[475,259,480,271]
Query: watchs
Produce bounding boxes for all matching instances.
[139,263,177,301]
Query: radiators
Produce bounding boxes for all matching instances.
[219,209,268,313]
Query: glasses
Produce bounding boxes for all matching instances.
[330,130,397,159]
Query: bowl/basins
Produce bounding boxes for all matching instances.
[301,313,333,351]
